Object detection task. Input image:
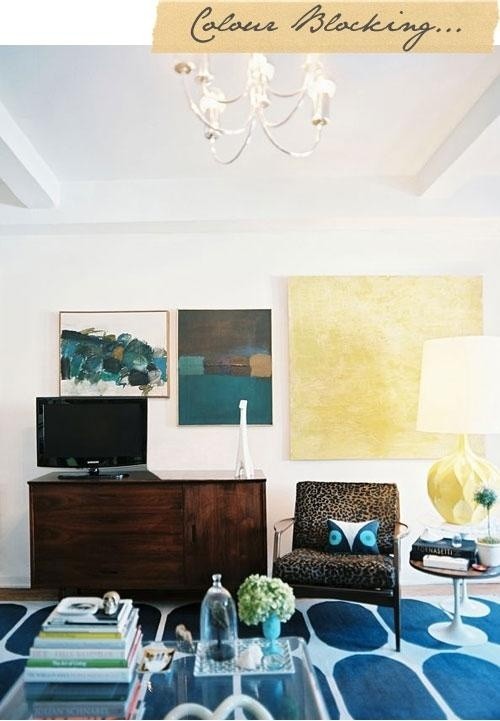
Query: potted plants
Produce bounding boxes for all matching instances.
[472,485,500,568]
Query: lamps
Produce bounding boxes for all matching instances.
[165,50,335,167]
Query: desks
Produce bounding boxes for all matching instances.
[1,634,329,719]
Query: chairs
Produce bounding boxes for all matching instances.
[274,479,411,654]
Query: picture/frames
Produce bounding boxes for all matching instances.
[56,308,172,400]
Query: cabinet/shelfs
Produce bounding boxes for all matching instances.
[26,468,268,594]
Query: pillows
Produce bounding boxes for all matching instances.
[326,517,383,555]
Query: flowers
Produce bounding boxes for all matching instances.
[235,574,297,625]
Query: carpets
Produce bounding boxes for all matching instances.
[0,587,499,720]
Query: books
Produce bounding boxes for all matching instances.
[22,594,150,720]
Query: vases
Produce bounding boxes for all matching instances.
[263,617,284,639]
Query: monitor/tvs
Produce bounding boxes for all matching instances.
[37,397,147,479]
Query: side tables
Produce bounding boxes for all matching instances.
[408,554,498,649]
